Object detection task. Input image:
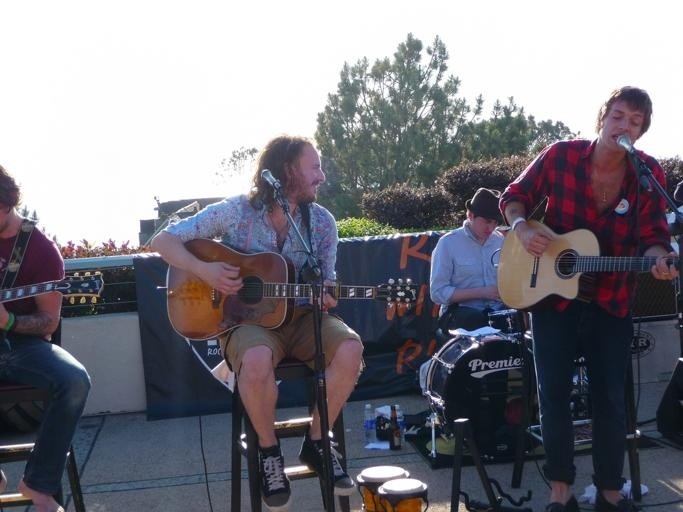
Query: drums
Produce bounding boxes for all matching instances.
[424,332,543,459]
[377,478,428,512]
[488,309,526,333]
[356,465,410,512]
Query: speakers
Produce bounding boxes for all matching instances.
[631,271,677,323]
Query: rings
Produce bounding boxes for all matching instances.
[662,271,668,276]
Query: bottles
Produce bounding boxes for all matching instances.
[361,403,376,444]
[385,404,402,449]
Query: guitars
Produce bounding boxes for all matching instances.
[156,237,420,341]
[497,219,683,311]
[0,271,105,305]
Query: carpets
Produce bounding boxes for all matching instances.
[404,401,669,471]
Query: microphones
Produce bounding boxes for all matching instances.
[615,135,646,164]
[261,169,283,190]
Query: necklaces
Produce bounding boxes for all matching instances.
[598,185,612,203]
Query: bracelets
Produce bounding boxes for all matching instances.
[3,311,18,333]
[511,217,525,232]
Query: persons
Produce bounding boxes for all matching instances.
[0,165,90,511]
[497,88,681,511]
[428,185,507,346]
[152,134,365,510]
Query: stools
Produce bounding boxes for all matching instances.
[512,327,643,502]
[0,379,88,512]
[221,351,349,511]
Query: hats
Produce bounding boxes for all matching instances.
[464,186,507,222]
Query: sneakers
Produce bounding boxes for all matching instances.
[297,421,359,498]
[257,434,295,512]
[588,487,643,512]
[543,491,581,511]
